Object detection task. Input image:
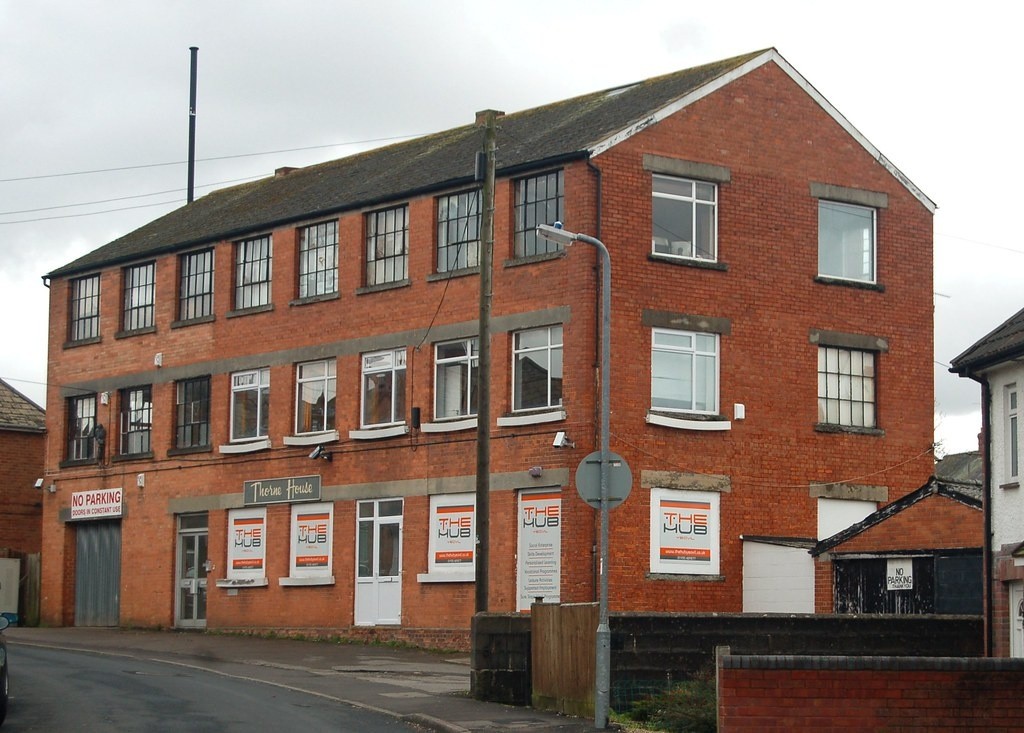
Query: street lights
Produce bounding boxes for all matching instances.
[535,219,633,731]
[468,109,508,703]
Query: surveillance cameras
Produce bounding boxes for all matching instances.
[308,445,333,462]
[553,431,576,449]
[34,478,44,489]
